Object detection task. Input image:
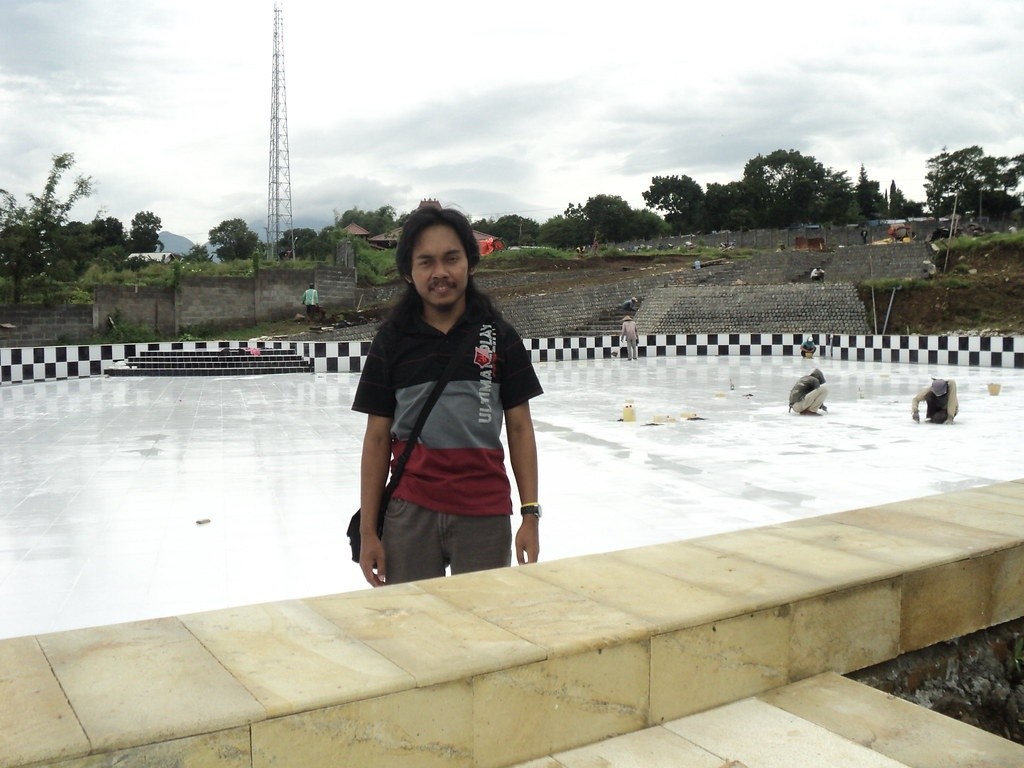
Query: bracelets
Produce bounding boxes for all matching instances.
[522,502,539,507]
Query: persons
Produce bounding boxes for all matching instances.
[594,241,598,255]
[576,245,586,253]
[623,297,638,311]
[1008,225,1018,234]
[810,266,825,282]
[923,257,936,281]
[800,336,816,358]
[719,241,727,250]
[684,241,693,250]
[657,243,664,250]
[351,207,544,589]
[722,239,736,252]
[911,377,959,424]
[789,367,827,417]
[776,242,785,253]
[301,283,319,322]
[278,252,284,260]
[621,315,640,360]
[861,227,868,244]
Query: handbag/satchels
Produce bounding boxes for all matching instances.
[347,486,386,569]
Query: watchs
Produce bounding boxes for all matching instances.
[521,504,542,519]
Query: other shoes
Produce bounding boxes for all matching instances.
[801,409,822,417]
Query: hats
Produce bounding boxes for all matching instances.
[932,379,947,397]
[810,368,827,385]
[808,336,814,341]
[623,315,633,321]
